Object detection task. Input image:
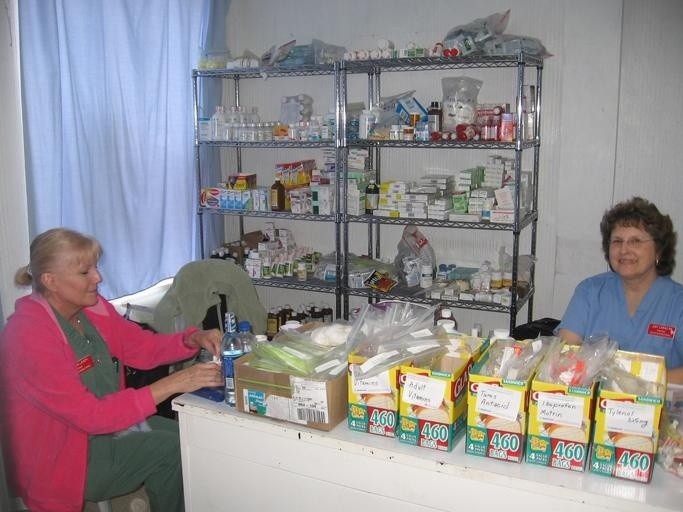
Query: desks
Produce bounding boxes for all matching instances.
[170,385,682,511]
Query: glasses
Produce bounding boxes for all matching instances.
[606,235,657,248]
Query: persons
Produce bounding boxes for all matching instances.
[0,228,223,511]
[551,197,682,387]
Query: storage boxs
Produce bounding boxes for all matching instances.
[236,322,349,433]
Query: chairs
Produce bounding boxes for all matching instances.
[153,259,267,336]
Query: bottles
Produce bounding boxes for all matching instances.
[420,261,516,310]
[347,79,519,145]
[200,226,321,286]
[195,91,338,145]
[268,179,286,212]
[220,312,258,410]
[363,179,381,215]
[345,37,393,60]
